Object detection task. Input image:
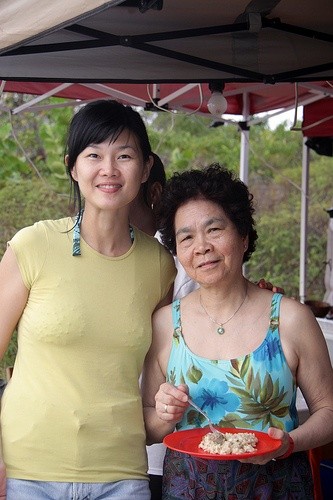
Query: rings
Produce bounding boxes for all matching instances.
[272,457,276,461]
[165,404,168,413]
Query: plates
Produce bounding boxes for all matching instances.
[163,427,282,461]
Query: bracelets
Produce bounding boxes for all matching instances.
[276,438,293,459]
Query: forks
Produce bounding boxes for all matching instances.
[188,398,224,436]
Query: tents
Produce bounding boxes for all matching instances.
[0,0,333,304]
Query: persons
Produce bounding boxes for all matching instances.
[1,98,294,500]
[140,165,333,500]
[129,153,201,299]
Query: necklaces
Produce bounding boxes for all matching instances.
[200,276,248,334]
